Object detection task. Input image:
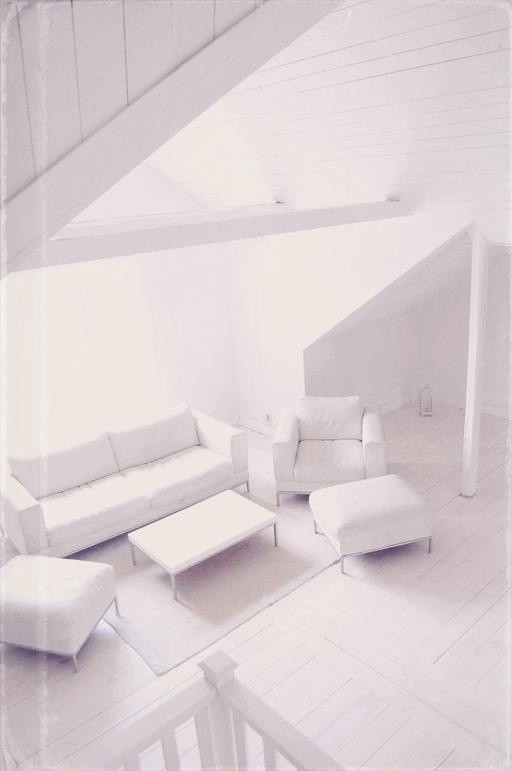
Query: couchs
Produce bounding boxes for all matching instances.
[0,403,249,558]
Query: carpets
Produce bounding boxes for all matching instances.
[65,492,341,675]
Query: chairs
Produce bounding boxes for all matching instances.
[270,395,387,508]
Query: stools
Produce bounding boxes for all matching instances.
[308,473,434,573]
[0,556,119,672]
